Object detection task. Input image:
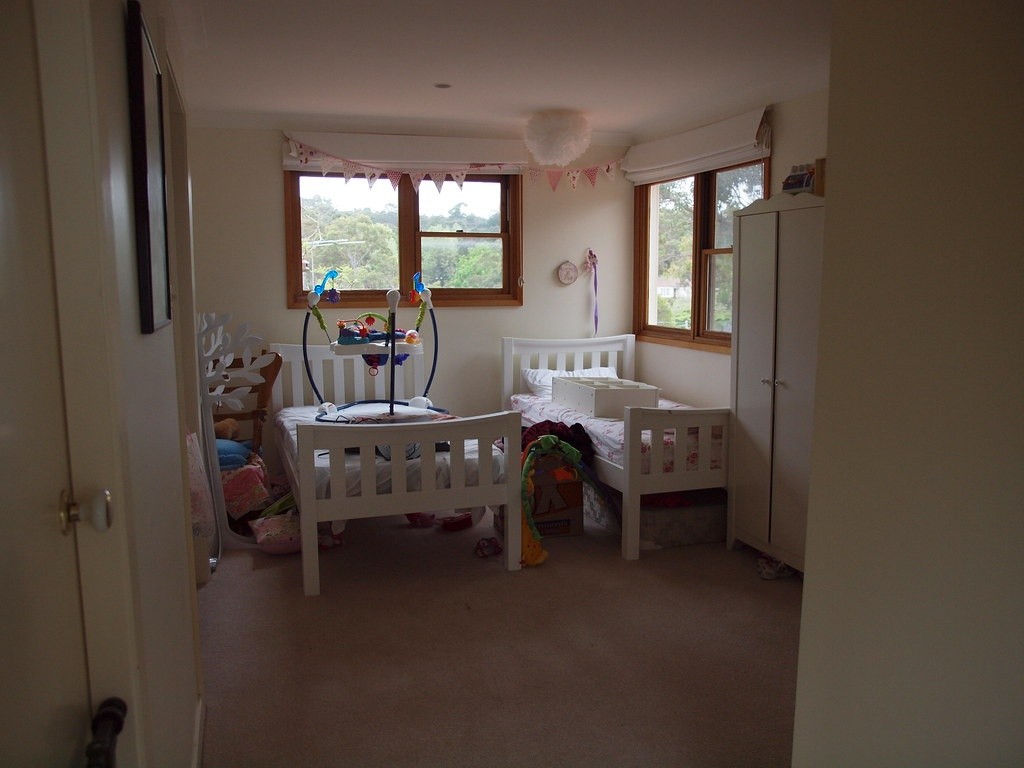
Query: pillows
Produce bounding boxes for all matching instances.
[520,366,618,397]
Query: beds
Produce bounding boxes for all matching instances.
[501,334,731,561]
[265,341,523,595]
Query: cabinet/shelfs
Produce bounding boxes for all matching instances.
[733,192,821,571]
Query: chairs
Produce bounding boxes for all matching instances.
[205,349,283,452]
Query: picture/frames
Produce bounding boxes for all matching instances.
[125,0,173,335]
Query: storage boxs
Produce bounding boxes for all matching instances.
[551,376,660,418]
[494,476,583,538]
